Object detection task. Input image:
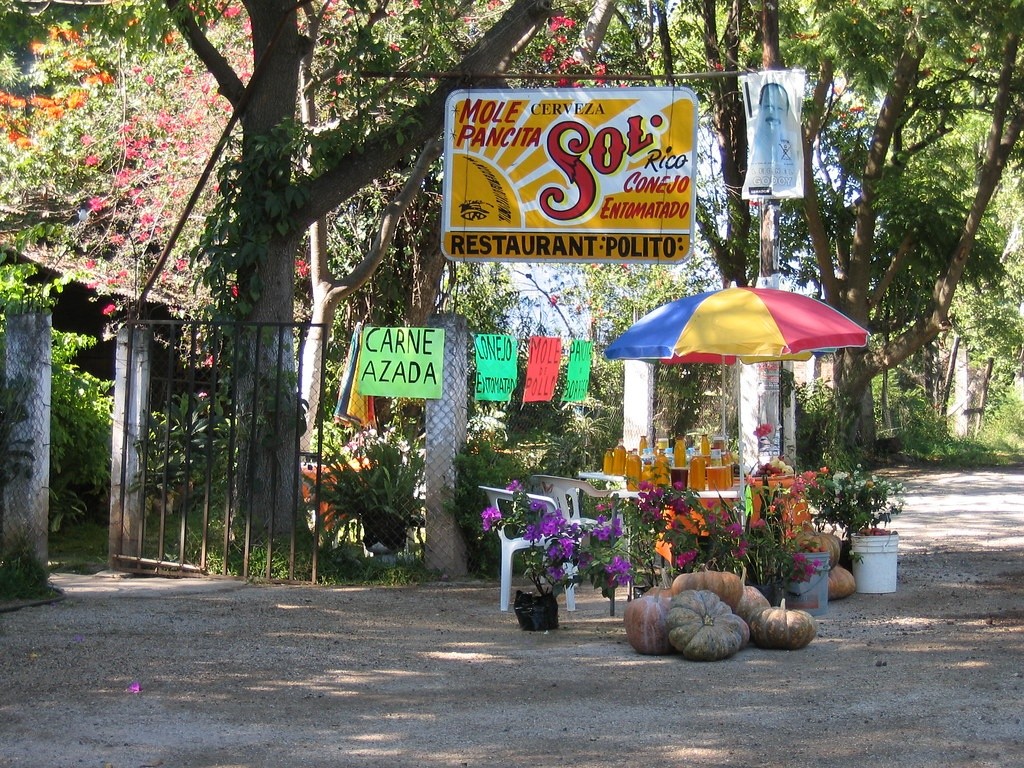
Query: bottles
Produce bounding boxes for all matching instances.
[603,434,734,491]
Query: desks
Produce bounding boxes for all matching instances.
[578,469,812,615]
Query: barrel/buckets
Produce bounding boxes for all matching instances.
[783,552,831,616]
[748,453,800,521]
[851,533,900,593]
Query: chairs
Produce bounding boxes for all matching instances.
[478,475,617,611]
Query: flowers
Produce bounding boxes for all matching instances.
[481,423,907,595]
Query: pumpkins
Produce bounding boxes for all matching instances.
[796,520,856,600]
[624,562,819,661]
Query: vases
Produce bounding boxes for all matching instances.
[755,584,784,607]
[514,590,558,631]
[838,540,854,576]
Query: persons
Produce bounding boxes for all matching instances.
[751,82,792,164]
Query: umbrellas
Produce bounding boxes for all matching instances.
[602,286,871,533]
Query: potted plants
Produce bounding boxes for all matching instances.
[301,441,426,564]
